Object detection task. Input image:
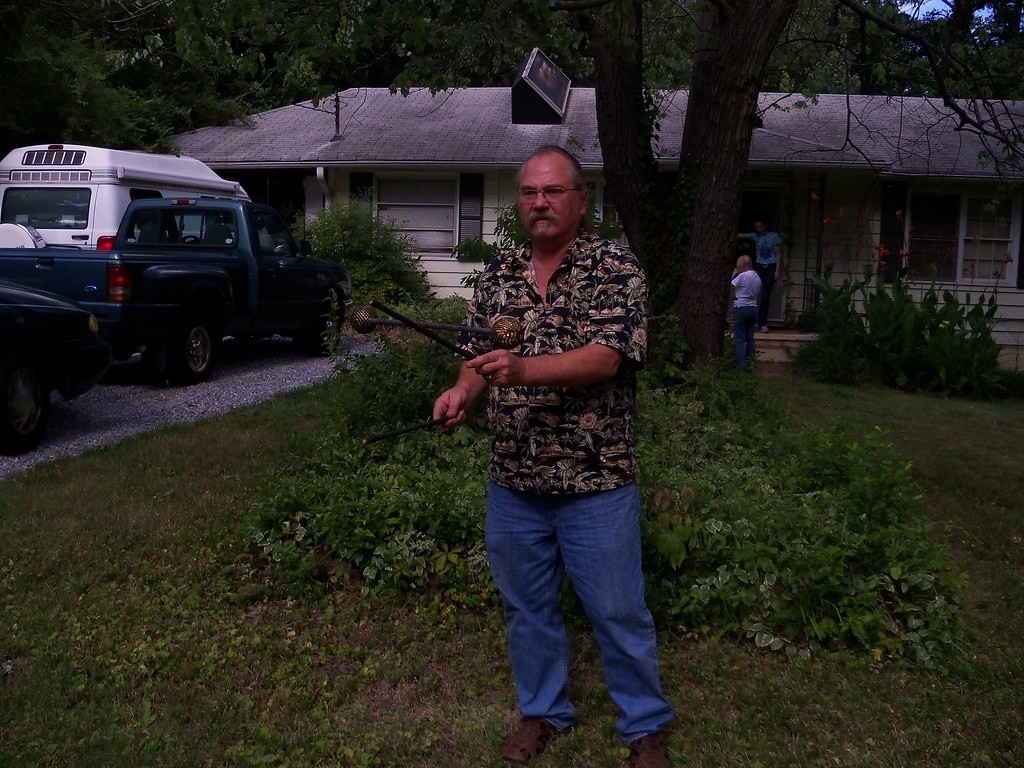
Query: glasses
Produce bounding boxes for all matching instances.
[514,185,580,203]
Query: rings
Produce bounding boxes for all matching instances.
[484,373,492,380]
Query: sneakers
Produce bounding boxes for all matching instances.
[499,714,552,762]
[628,734,670,767]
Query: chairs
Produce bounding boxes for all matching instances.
[203,223,233,244]
[135,224,168,242]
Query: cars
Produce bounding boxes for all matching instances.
[0,277,108,457]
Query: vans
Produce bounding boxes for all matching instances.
[0,143,258,258]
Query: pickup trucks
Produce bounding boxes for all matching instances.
[1,197,349,389]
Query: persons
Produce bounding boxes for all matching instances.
[738,219,782,333]
[730,255,762,373]
[432,145,677,766]
